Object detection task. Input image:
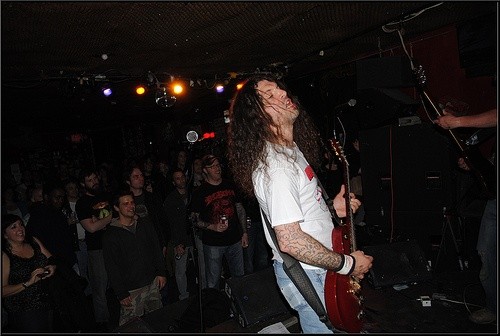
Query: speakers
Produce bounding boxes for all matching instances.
[115,267,296,333]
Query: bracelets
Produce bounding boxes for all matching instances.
[335,255,356,275]
[23,284,28,288]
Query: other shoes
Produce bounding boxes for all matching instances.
[95,321,109,332]
[469,307,496,325]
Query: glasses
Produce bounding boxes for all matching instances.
[205,163,223,169]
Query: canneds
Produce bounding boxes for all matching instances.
[246,217,252,228]
[220,214,228,229]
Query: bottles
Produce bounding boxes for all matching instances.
[219,212,228,228]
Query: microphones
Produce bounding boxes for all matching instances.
[334,99,357,111]
[186,131,199,172]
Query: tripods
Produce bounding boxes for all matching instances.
[432,207,465,273]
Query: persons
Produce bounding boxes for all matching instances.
[436,109,497,324]
[3,149,272,332]
[227,75,373,334]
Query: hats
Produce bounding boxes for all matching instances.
[200,154,218,168]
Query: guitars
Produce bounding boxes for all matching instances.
[324,137,365,333]
